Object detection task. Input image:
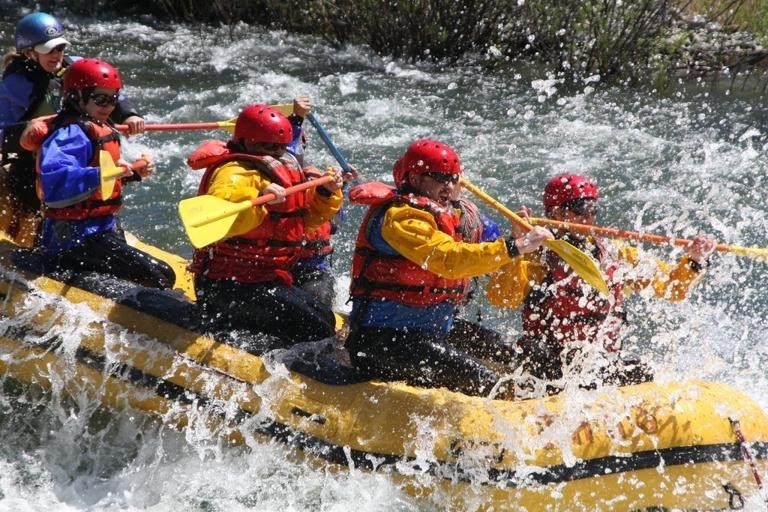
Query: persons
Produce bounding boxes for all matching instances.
[482,173,717,397]
[185,95,358,370]
[345,139,554,399]
[1,12,175,291]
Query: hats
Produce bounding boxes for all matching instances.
[29,36,72,54]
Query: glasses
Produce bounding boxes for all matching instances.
[560,199,599,215]
[49,43,66,54]
[425,172,460,185]
[85,93,119,108]
[263,144,287,151]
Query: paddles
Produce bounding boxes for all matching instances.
[460,178,608,294]
[115,105,310,132]
[177,167,338,250]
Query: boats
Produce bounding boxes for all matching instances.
[1,196,768,512]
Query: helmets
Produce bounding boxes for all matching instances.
[543,173,600,212]
[64,58,121,90]
[400,139,460,181]
[15,12,63,50]
[233,104,294,146]
